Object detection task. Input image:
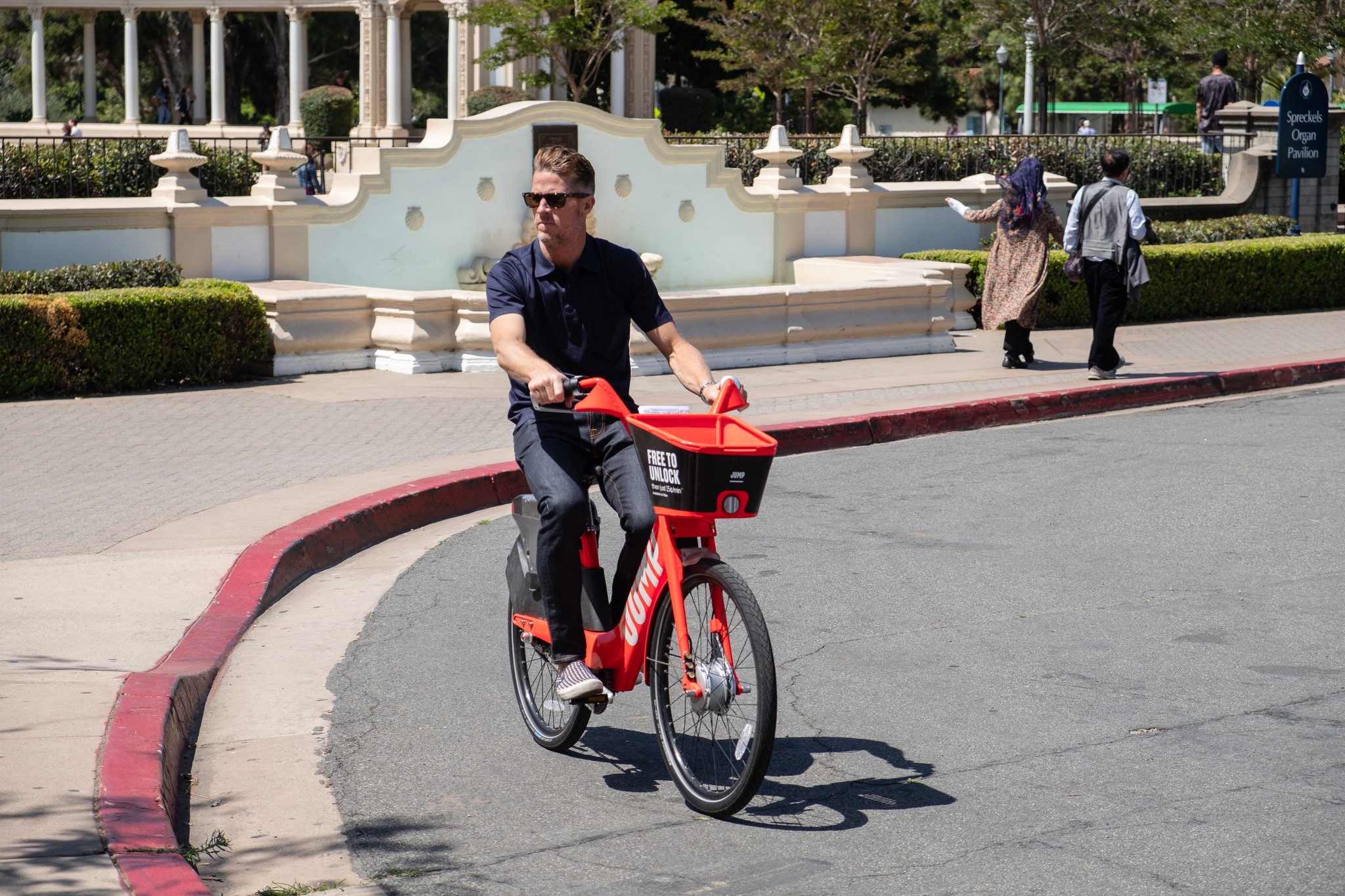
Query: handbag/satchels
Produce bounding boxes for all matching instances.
[1063,245,1086,282]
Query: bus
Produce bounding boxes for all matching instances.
[1016,101,1197,136]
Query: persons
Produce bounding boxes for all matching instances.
[175,85,196,122]
[1065,146,1152,383]
[943,153,1067,371]
[1194,45,1238,155]
[1076,119,1096,136]
[62,118,83,142]
[296,142,321,197]
[151,76,173,124]
[487,147,748,706]
[257,119,272,152]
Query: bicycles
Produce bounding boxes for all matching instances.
[504,370,778,820]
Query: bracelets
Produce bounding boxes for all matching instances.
[695,377,717,400]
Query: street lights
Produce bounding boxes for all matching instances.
[995,44,1009,135]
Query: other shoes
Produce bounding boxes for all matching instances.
[1113,355,1125,374]
[635,673,642,685]
[1024,342,1034,364]
[555,658,606,699]
[1087,366,1116,379]
[1002,356,1029,369]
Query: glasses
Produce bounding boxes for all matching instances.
[522,190,587,209]
